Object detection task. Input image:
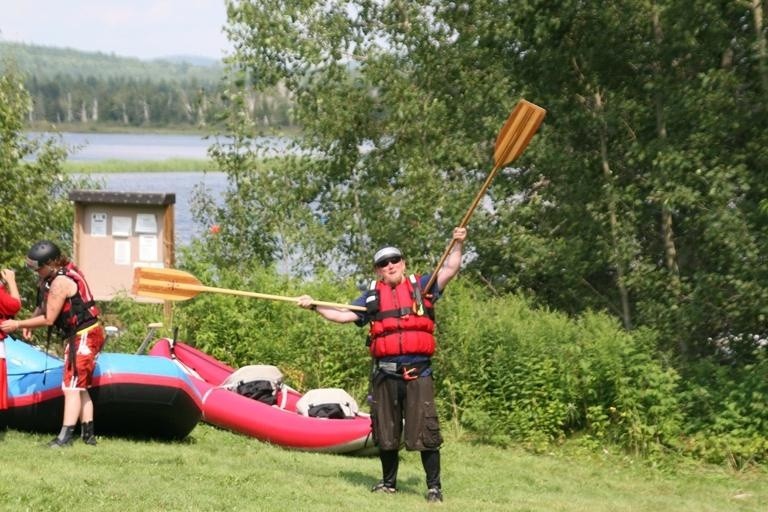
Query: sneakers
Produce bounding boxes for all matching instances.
[45,438,98,448]
[372,482,398,495]
[426,486,444,503]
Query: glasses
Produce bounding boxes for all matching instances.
[378,258,401,267]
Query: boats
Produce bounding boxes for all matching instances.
[148,334,406,458]
[1,335,204,440]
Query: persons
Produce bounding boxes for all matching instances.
[296,226,467,504]
[0,240,106,449]
[0,268,22,432]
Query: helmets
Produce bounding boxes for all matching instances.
[27,242,61,270]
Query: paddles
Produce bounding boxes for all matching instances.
[423,98,546,293]
[131,267,367,310]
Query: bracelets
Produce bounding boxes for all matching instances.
[311,305,316,311]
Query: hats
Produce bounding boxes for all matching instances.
[372,245,402,265]
